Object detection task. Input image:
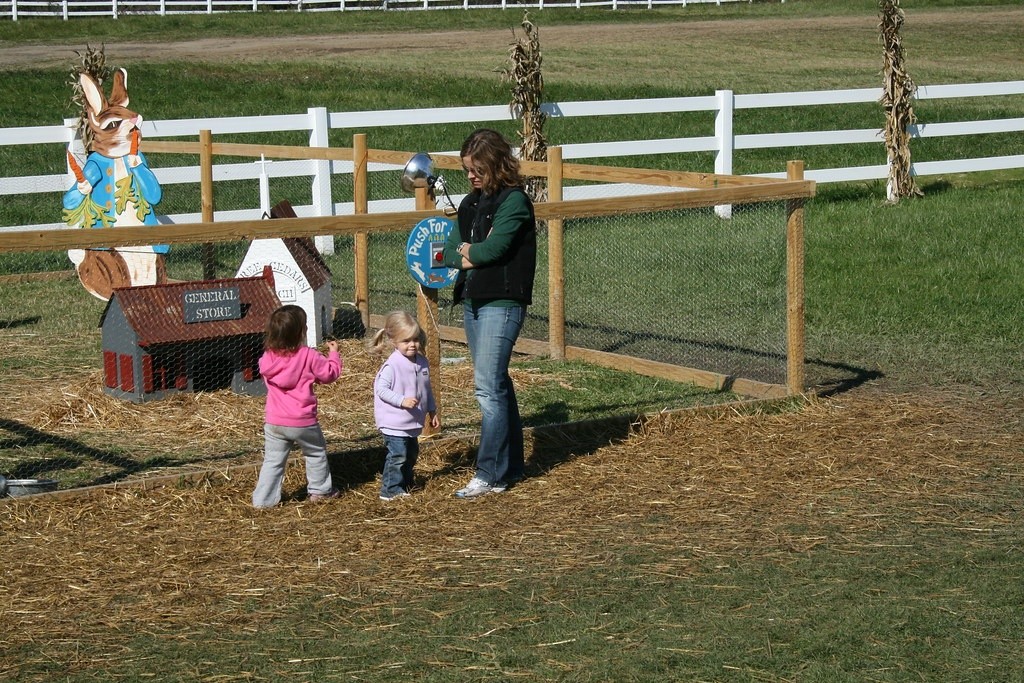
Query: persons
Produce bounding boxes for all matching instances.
[370,310,440,500]
[441,128,536,497]
[252,305,342,509]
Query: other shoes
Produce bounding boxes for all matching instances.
[310,489,339,503]
[380,483,418,501]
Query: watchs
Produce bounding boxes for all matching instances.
[458,242,468,252]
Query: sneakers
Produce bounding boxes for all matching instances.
[457,477,506,496]
